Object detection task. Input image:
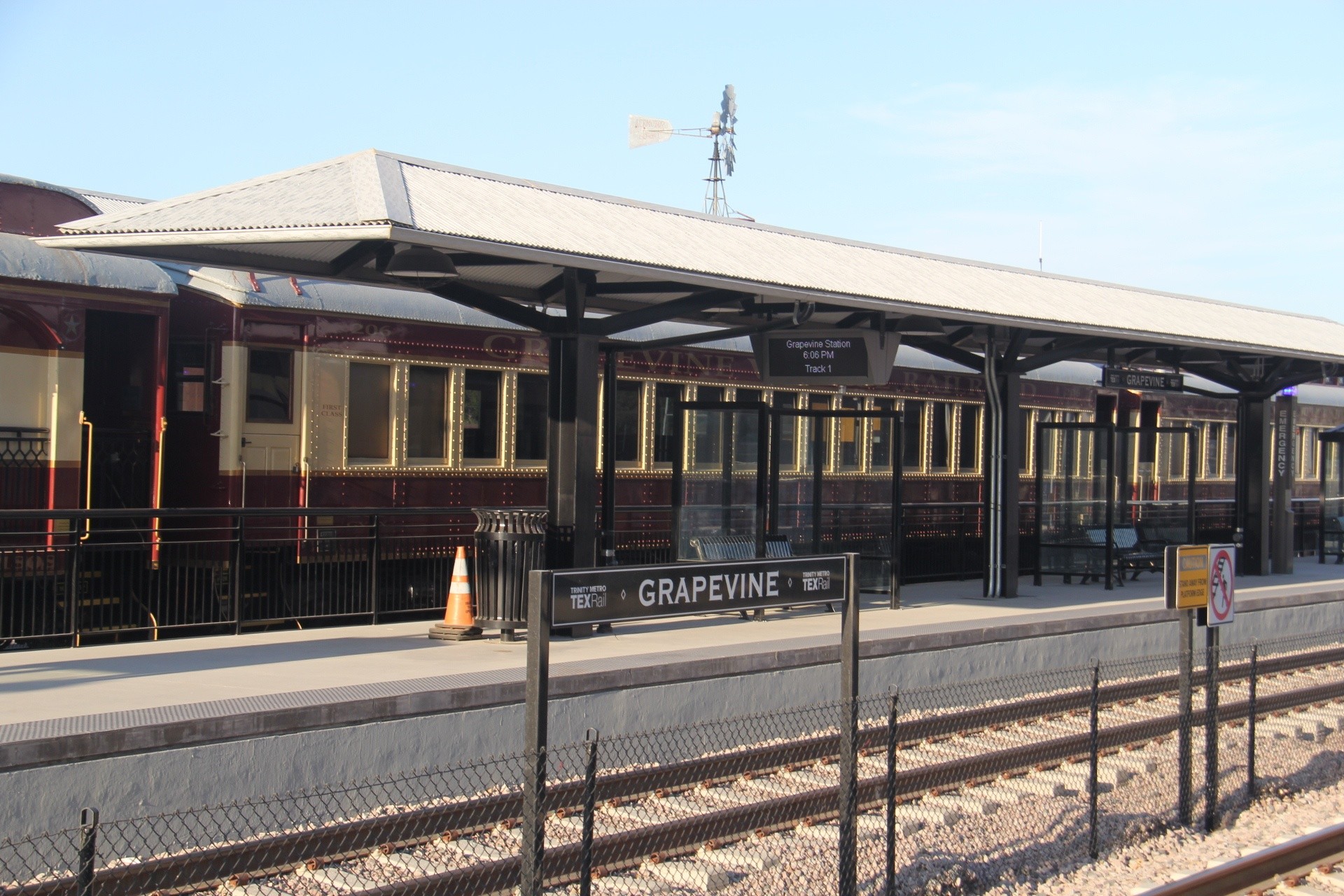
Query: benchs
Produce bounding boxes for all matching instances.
[1065,523,1169,587]
[688,534,835,621]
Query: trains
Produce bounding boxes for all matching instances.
[1,230,1343,652]
